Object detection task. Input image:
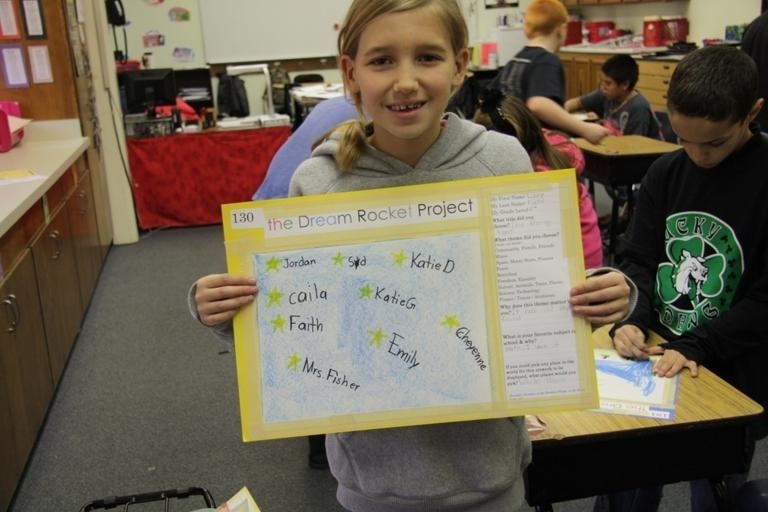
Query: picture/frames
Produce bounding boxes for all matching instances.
[1,0,56,90]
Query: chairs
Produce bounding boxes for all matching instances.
[603,112,677,248]
[294,74,325,118]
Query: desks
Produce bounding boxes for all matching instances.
[565,125,693,253]
[290,82,351,119]
[511,318,762,511]
[125,120,294,227]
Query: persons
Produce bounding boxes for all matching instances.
[493,0,611,144]
[444,85,603,271]
[188,1,637,511]
[250,92,375,199]
[606,46,768,510]
[739,8,768,135]
[563,52,666,143]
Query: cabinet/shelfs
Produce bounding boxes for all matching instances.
[64,166,103,320]
[30,201,84,390]
[557,52,612,102]
[636,60,682,113]
[0,247,56,512]
